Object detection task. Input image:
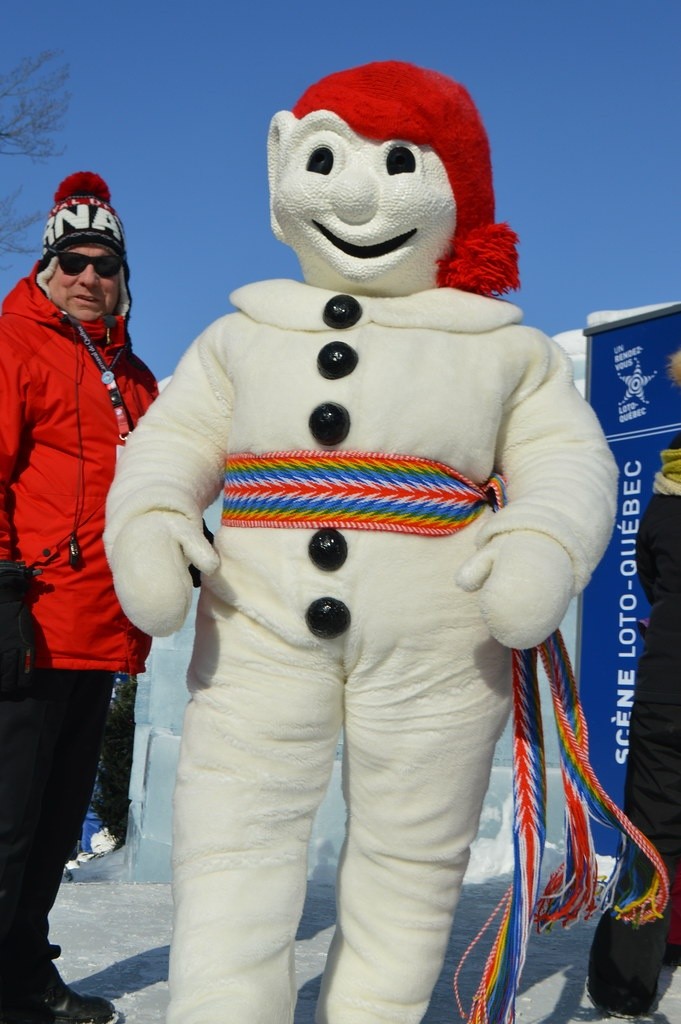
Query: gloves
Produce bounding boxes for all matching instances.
[0,570,34,692]
[188,517,215,589]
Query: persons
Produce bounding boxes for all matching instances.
[0,170,213,1024]
[103,62,621,1024]
[567,346,681,1024]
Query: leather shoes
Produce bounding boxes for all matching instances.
[48,944,61,961]
[0,961,115,1024]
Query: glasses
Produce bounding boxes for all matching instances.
[46,246,124,278]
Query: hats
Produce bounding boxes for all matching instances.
[34,170,132,322]
